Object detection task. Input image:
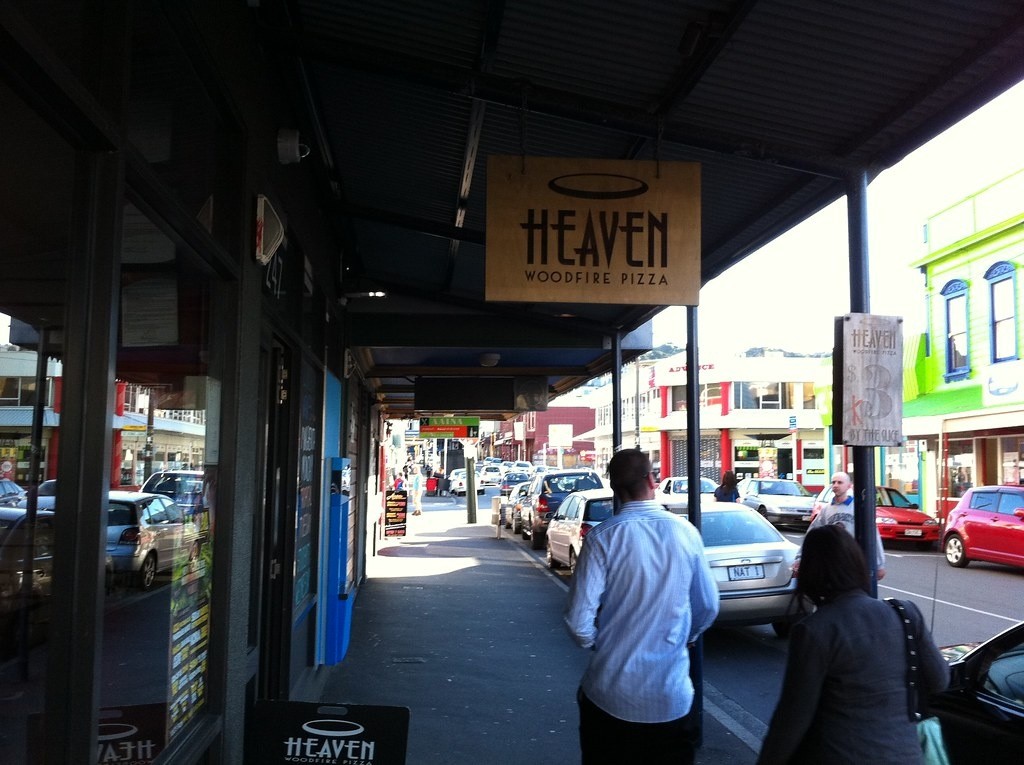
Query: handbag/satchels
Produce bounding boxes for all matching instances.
[913,713,948,765]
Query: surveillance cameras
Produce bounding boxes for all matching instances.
[277,128,311,165]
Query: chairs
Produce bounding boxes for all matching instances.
[575,480,591,490]
[702,521,756,542]
[549,483,560,492]
[592,506,607,519]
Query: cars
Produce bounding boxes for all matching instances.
[733,475,821,530]
[810,487,935,544]
[0,475,62,510]
[543,487,612,575]
[501,473,528,528]
[1,506,115,619]
[138,469,206,550]
[449,457,530,495]
[518,469,603,553]
[941,484,1024,568]
[913,620,1023,765]
[105,490,203,587]
[655,474,717,503]
[663,502,802,640]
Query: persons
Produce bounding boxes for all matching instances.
[714,471,740,503]
[791,472,886,611]
[411,466,423,516]
[754,522,951,765]
[564,449,720,765]
[389,462,432,489]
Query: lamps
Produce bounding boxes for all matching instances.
[255,194,285,268]
[479,353,501,367]
[343,347,357,380]
